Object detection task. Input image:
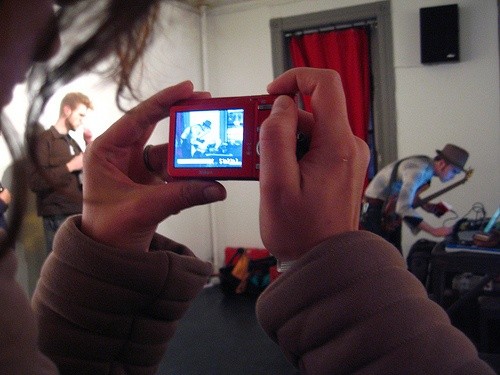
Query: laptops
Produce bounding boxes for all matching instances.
[452,207,500,242]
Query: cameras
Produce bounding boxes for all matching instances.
[167,95,299,180]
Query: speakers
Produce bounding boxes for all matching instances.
[420,3,460,64]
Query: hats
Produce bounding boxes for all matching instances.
[436,143,470,174]
[203,120,211,128]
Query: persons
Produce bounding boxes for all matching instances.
[0,0,500,375]
[359,144,470,259]
[21,93,93,254]
[178,120,211,159]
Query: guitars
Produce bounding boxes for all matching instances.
[383,168,475,231]
